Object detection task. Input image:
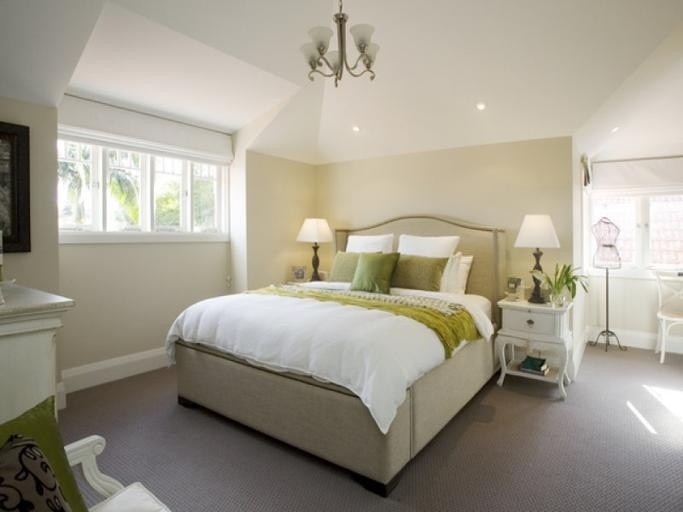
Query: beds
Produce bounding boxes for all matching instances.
[171,215,507,499]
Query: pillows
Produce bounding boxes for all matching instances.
[327,233,475,296]
[0,390,87,512]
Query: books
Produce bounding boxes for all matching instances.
[519,355,550,376]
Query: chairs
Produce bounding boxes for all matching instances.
[650,269,682,365]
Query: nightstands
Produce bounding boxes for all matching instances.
[493,286,577,399]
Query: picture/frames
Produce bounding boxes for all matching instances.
[0,120,35,254]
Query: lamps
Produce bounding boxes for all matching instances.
[295,216,334,283]
[513,214,559,303]
[301,1,381,88]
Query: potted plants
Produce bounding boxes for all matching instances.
[544,261,591,308]
